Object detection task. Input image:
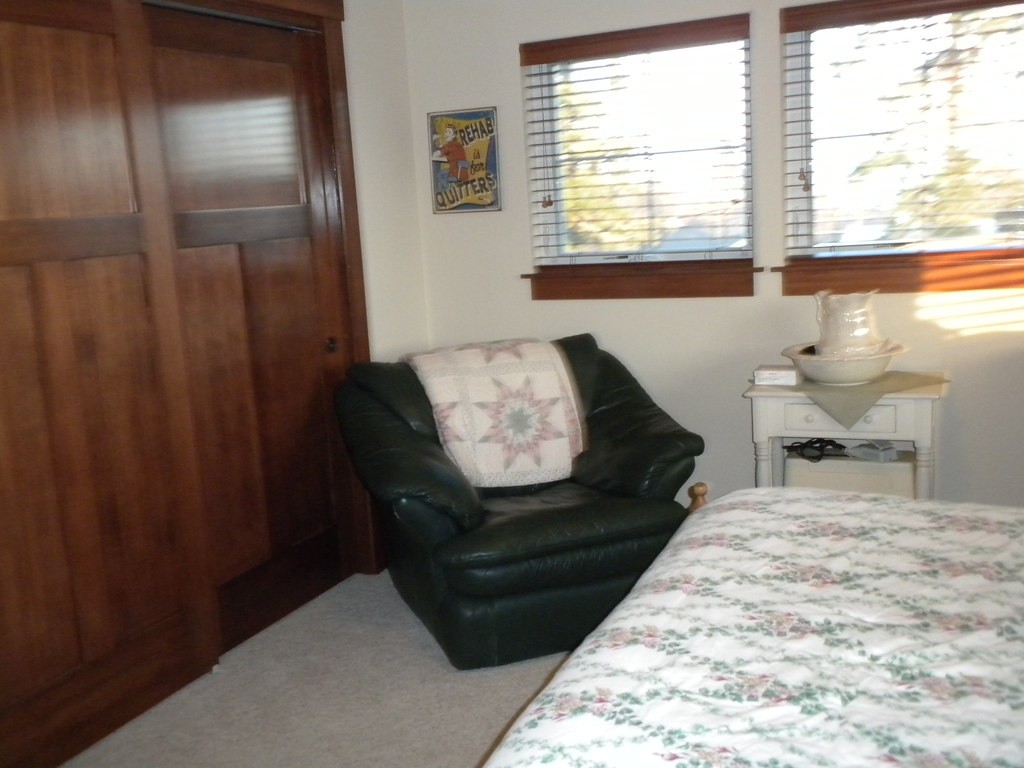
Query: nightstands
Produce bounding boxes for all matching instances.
[742,372,951,500]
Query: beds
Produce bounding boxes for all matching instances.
[481,490,1023,768]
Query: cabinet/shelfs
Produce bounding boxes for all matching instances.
[1,1,389,768]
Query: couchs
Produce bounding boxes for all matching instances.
[332,333,705,672]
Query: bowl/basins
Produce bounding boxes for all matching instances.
[781,341,904,388]
